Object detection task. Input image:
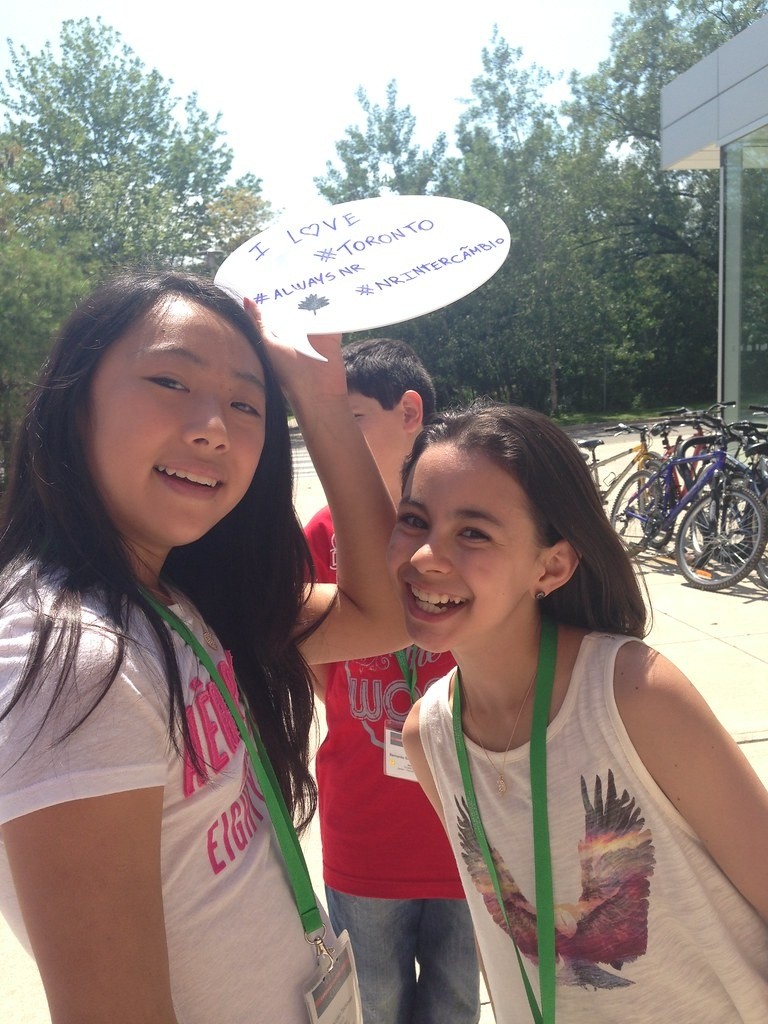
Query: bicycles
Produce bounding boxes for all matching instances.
[575,400,768,591]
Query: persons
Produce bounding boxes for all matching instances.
[386,404,766,1024]
[2,271,417,1024]
[296,335,482,1024]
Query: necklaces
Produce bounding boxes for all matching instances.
[461,675,539,797]
